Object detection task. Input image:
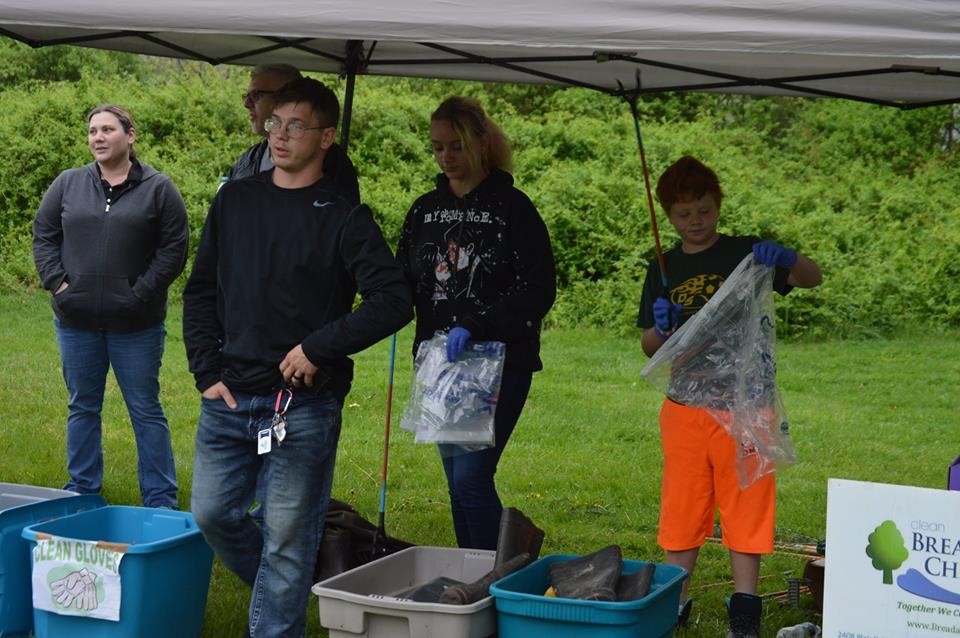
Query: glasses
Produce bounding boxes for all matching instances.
[265,119,330,138]
[242,90,272,102]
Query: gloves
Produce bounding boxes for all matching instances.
[754,243,797,270]
[654,297,681,331]
[447,328,472,360]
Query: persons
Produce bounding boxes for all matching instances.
[216,63,360,206]
[182,76,414,638]
[32,106,190,511]
[395,96,556,551]
[636,156,822,638]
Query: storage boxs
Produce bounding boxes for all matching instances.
[488,553,690,638]
[0,482,214,638]
[311,545,498,638]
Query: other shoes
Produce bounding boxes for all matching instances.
[725,592,762,638]
[678,600,694,627]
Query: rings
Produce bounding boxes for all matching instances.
[293,374,301,380]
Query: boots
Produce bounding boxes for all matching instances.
[395,508,544,604]
[546,545,655,602]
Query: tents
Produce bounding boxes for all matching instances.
[0,1,960,153]
